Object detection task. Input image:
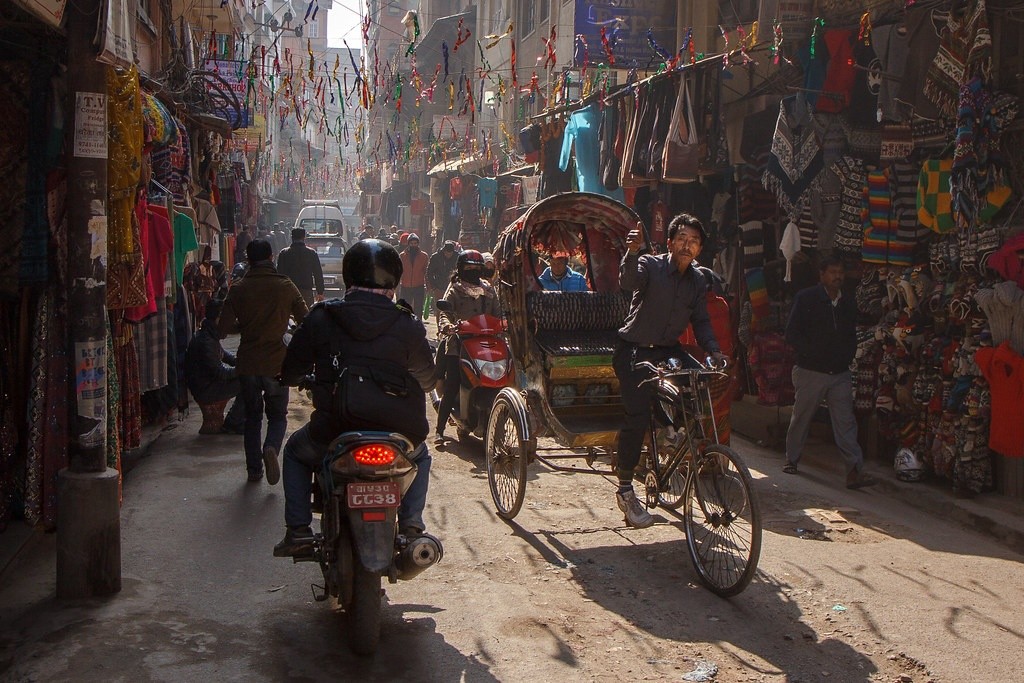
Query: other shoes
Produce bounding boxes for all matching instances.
[847,468,875,488]
[222,419,246,434]
[434,434,444,444]
[697,469,726,479]
[782,461,797,473]
[247,464,264,481]
[264,445,280,484]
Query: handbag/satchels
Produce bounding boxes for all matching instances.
[334,356,429,446]
[425,294,431,320]
[601,69,708,189]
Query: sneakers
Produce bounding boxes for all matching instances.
[615,486,654,529]
[663,435,699,457]
[274,524,313,556]
[397,528,419,547]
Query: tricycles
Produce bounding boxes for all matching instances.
[483,190,765,600]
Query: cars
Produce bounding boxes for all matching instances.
[303,231,350,290]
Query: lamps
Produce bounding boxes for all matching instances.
[270,20,278,32]
[284,12,293,22]
[295,26,304,37]
[517,123,542,153]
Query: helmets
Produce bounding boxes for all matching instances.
[457,250,485,284]
[342,237,404,291]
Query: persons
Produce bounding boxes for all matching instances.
[215,239,310,485]
[681,265,733,476]
[435,250,498,442]
[531,251,590,291]
[270,238,437,556]
[612,213,731,528]
[184,302,247,436]
[426,241,461,318]
[347,220,429,318]
[279,227,325,308]
[234,220,291,261]
[782,258,880,487]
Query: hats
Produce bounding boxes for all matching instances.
[480,252,495,266]
[847,221,1001,495]
[551,250,568,260]
[291,228,305,239]
[400,234,410,246]
[780,221,802,282]
[407,233,420,245]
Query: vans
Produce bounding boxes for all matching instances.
[289,199,351,247]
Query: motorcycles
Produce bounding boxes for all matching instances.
[271,330,444,658]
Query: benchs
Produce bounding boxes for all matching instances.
[525,289,631,357]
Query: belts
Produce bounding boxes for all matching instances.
[618,336,662,349]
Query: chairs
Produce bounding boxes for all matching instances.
[327,245,343,257]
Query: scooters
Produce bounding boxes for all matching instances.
[426,299,515,460]
